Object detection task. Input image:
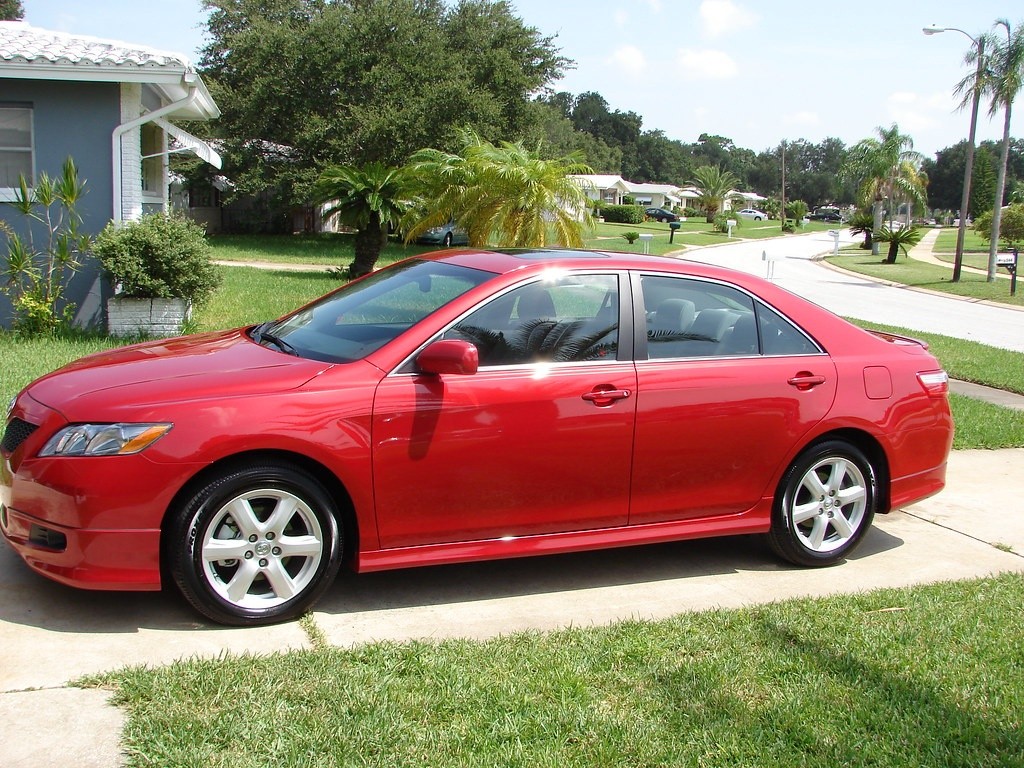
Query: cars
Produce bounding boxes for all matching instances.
[0,248,954,630]
[645,208,680,223]
[736,209,768,221]
[822,213,842,220]
[417,220,470,248]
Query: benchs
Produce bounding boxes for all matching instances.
[647,299,766,357]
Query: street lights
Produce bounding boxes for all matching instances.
[922,25,985,282]
[782,142,804,224]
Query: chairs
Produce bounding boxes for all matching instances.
[489,288,615,364]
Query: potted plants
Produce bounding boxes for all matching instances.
[86,203,224,337]
